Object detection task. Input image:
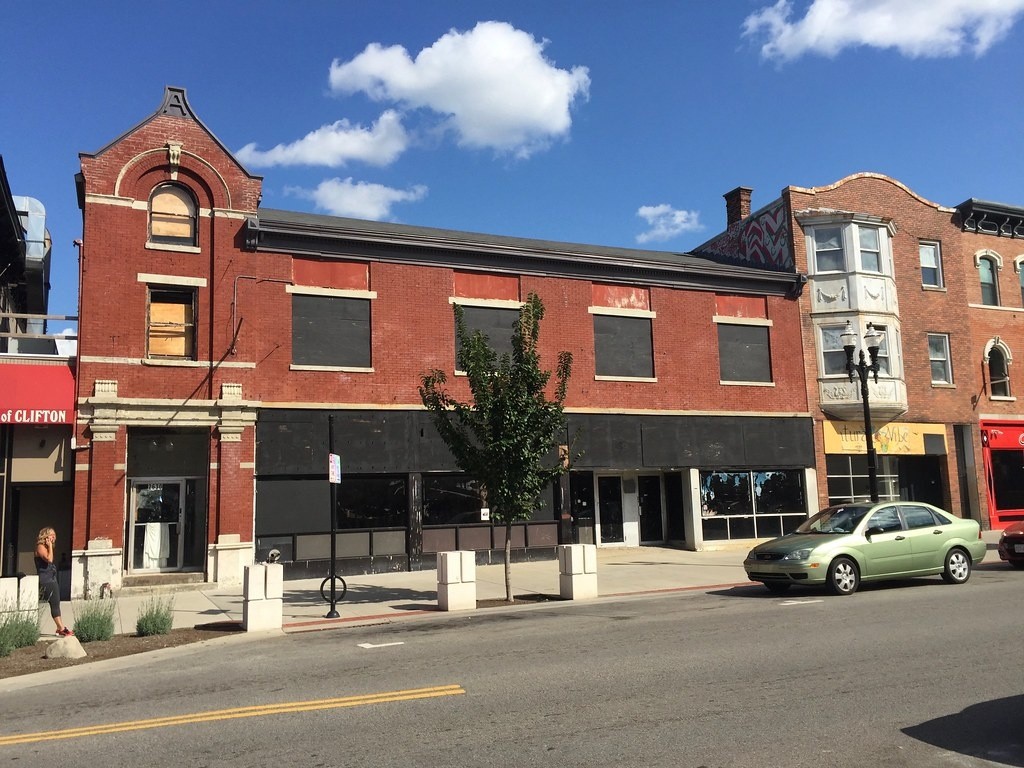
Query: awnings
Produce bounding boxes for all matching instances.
[0,364,75,425]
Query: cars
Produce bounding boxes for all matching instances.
[743,500,988,596]
[998,517,1024,567]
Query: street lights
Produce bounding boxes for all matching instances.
[839,319,881,504]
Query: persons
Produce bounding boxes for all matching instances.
[34,527,73,637]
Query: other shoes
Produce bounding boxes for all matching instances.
[55,628,75,637]
